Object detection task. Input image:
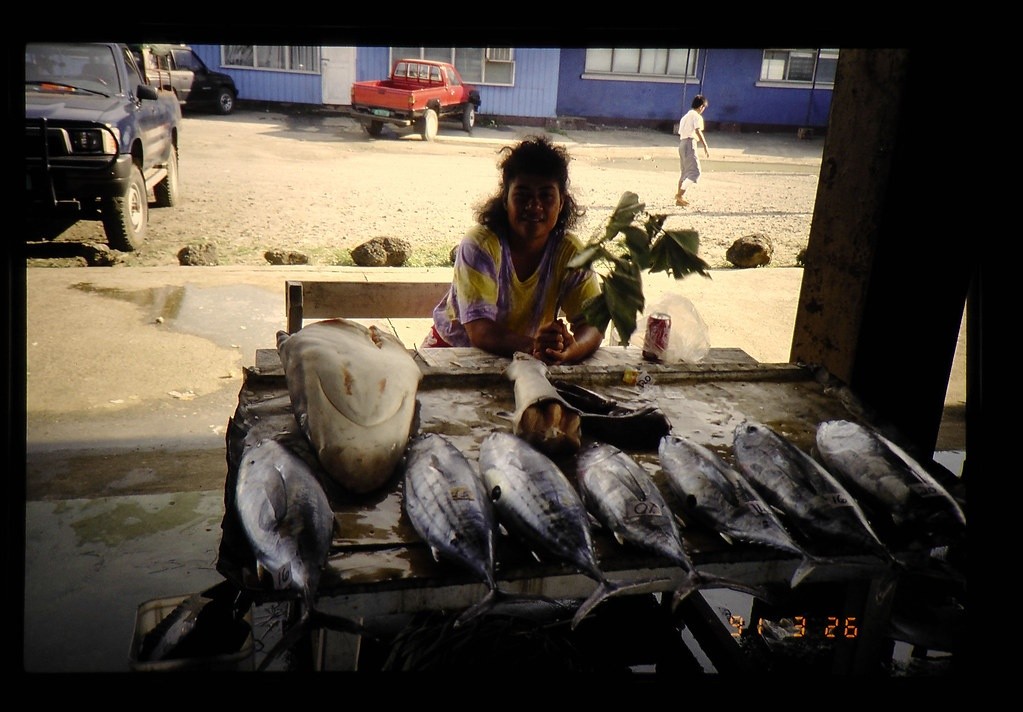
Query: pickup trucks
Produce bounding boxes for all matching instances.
[127,46,237,115]
[24,43,182,253]
[347,58,480,143]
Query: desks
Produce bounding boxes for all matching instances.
[241,360,974,712]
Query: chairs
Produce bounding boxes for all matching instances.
[25,61,39,76]
[81,64,115,81]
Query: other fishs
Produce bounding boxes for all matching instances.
[402,420,981,628]
[235,435,367,680]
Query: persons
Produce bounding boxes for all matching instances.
[426,138,608,365]
[675,96,708,205]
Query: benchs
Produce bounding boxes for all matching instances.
[284,278,452,336]
